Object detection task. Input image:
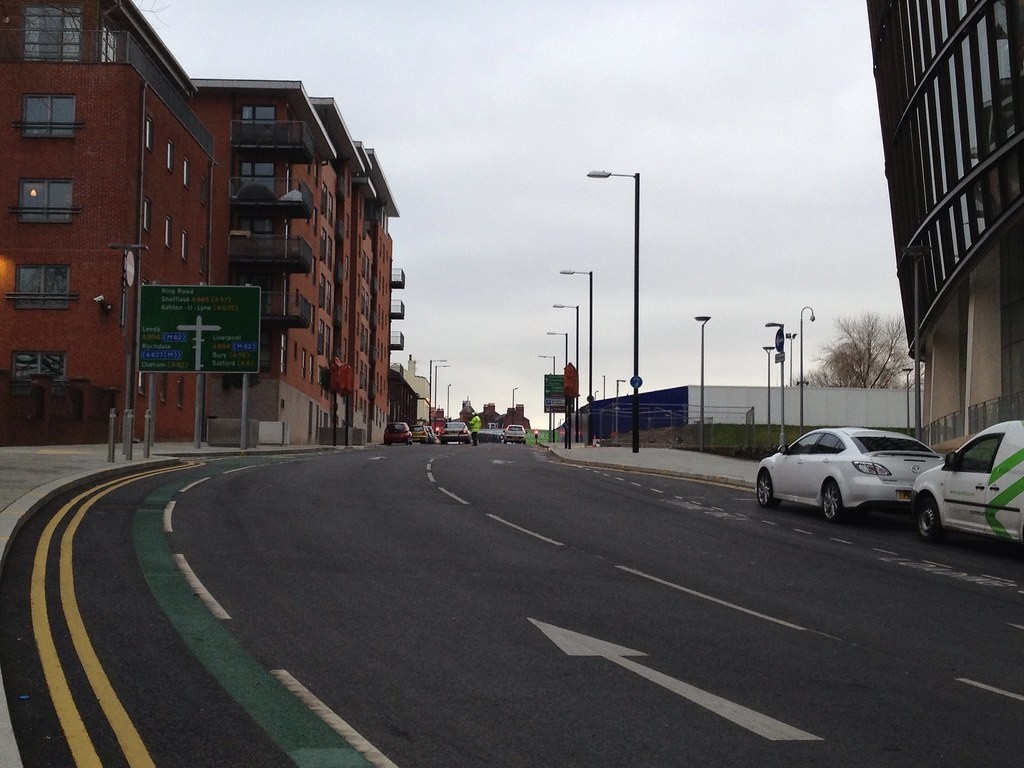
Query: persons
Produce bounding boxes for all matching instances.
[468,413,481,446]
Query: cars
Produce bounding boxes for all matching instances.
[384,422,414,446]
[755,426,950,523]
[439,421,471,445]
[409,424,436,444]
[908,419,1024,547]
[502,425,528,445]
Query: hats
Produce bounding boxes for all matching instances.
[471,411,478,415]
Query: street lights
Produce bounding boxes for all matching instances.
[692,316,711,451]
[433,363,451,429]
[558,269,593,444]
[428,359,448,425]
[614,380,626,442]
[586,170,642,453]
[447,384,452,425]
[512,387,518,425]
[764,322,787,448]
[602,374,606,404]
[798,306,816,436]
[594,391,599,403]
[902,368,913,436]
[546,331,572,449]
[554,305,579,444]
[762,346,776,449]
[538,355,555,444]
[786,332,799,386]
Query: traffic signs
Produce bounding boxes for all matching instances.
[136,285,262,374]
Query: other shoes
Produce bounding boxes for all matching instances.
[472,443,477,446]
